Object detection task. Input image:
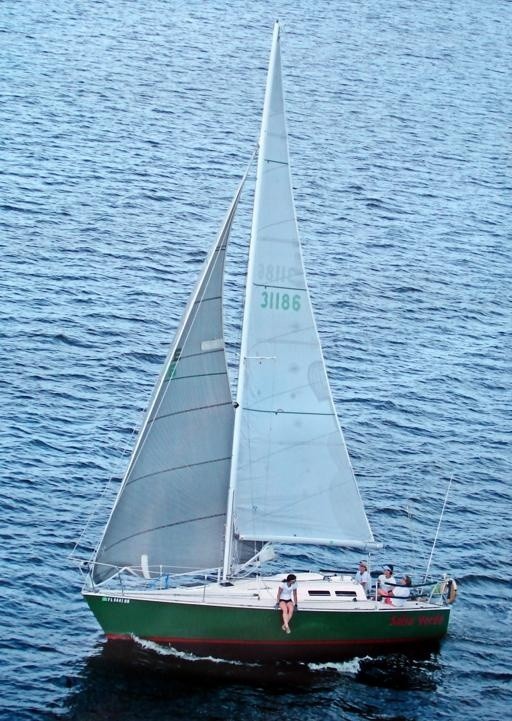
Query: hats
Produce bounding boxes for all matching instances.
[381,563,393,572]
[360,562,368,571]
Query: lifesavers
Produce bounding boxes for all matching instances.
[441,578,457,604]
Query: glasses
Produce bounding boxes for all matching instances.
[291,580,296,583]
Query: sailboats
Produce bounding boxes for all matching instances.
[69,20,456,661]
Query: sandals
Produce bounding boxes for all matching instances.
[282,625,291,634]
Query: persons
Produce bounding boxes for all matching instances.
[275,574,298,635]
[354,559,368,582]
[373,564,396,601]
[378,575,412,607]
[359,563,371,599]
